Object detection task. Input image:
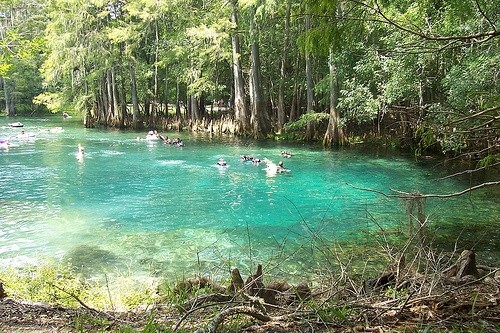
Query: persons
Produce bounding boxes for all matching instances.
[77,144,87,159]
[62,110,70,118]
[138,127,297,177]
[16,129,31,140]
